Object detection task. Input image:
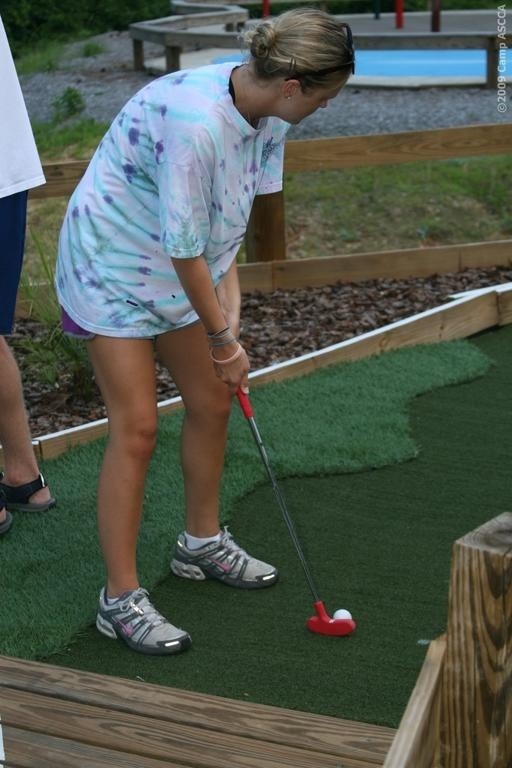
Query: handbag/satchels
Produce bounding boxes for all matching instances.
[210,343,242,365]
[207,326,235,346]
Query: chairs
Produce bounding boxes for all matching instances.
[96,587,193,655]
[170,526,278,589]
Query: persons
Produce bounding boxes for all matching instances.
[54,6,355,656]
[0,19,56,535]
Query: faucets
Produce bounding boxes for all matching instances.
[0,472,56,533]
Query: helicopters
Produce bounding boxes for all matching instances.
[332,608,352,621]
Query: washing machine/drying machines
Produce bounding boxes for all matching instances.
[235,386,356,637]
